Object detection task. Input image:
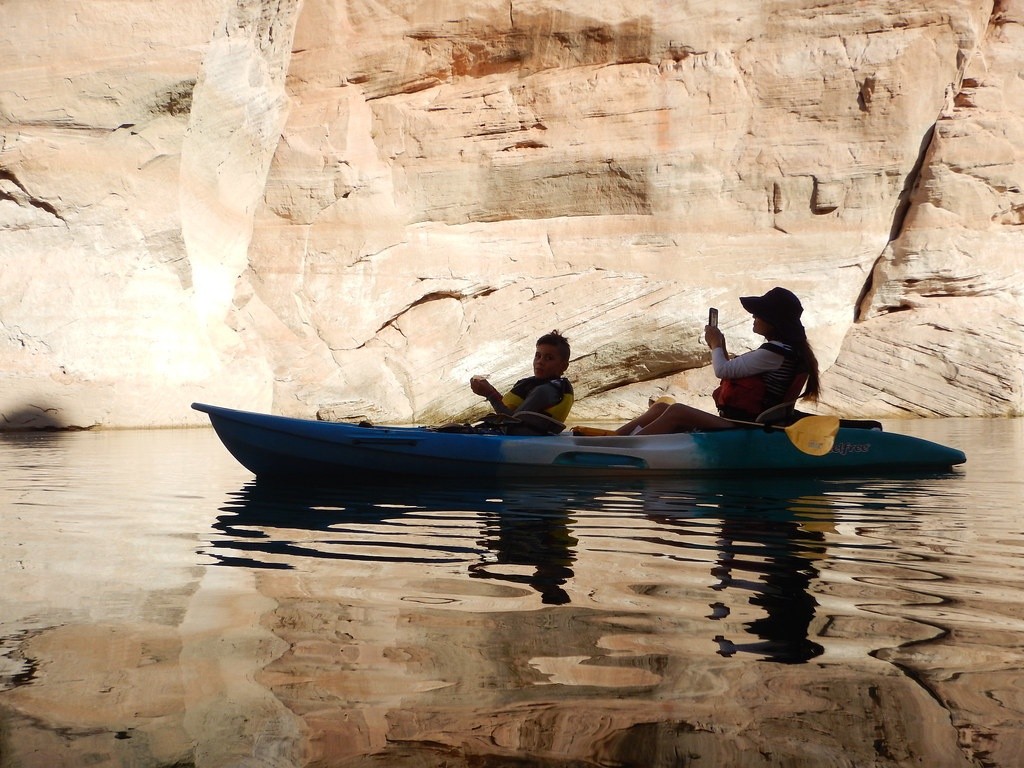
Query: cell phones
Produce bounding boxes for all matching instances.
[709,308,719,327]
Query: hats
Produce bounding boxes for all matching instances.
[740,287,804,327]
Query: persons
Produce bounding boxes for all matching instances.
[615,287,821,437]
[470,334,575,435]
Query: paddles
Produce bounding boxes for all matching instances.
[654,396,842,459]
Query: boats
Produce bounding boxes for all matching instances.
[189,393,969,494]
